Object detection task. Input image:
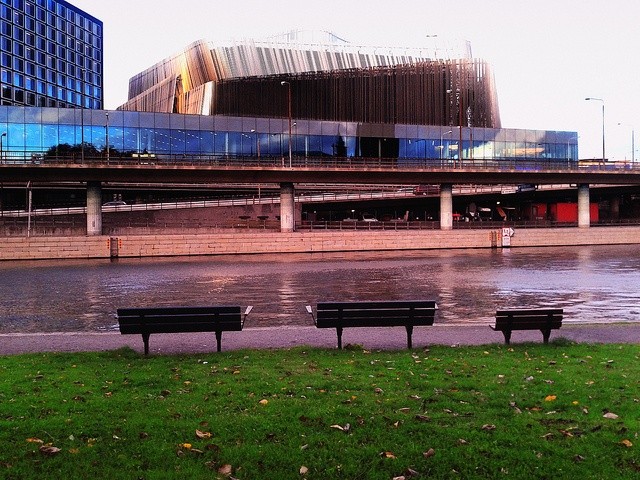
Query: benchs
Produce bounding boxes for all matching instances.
[117,305,253,356]
[306,300,438,350]
[489,309,563,344]
